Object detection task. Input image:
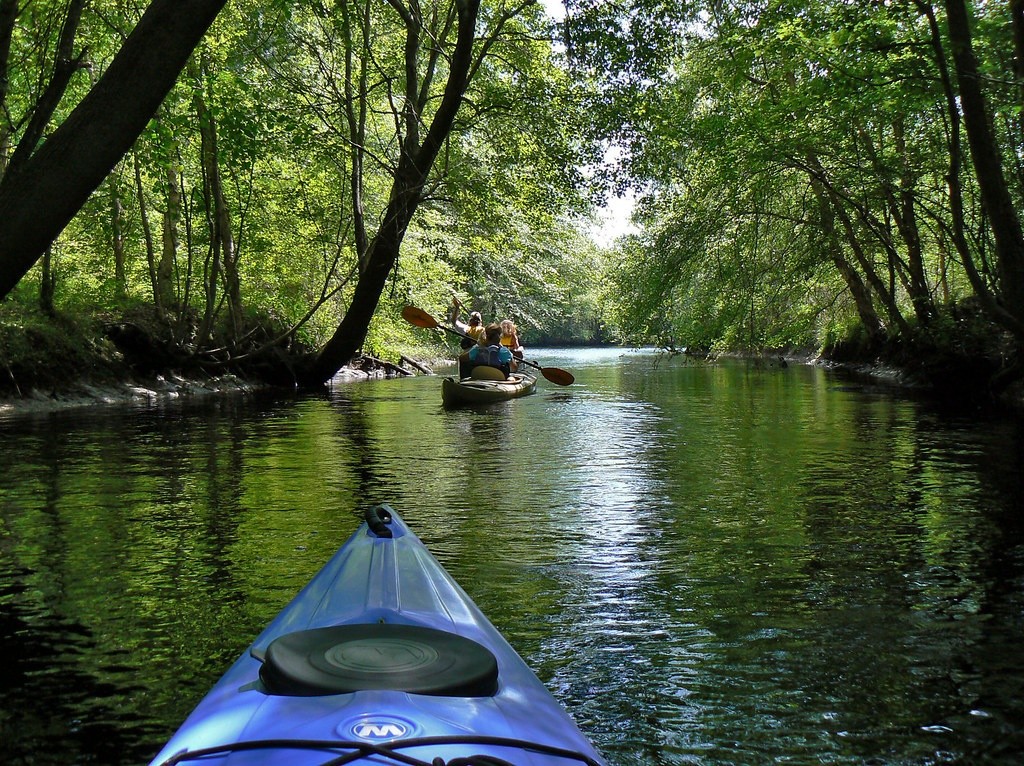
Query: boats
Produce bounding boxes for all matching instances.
[148,500,608,766]
[442,363,539,403]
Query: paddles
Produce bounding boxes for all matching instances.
[401,305,575,386]
[450,292,471,317]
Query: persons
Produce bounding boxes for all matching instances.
[459,323,518,381]
[452,297,487,344]
[500,320,523,366]
